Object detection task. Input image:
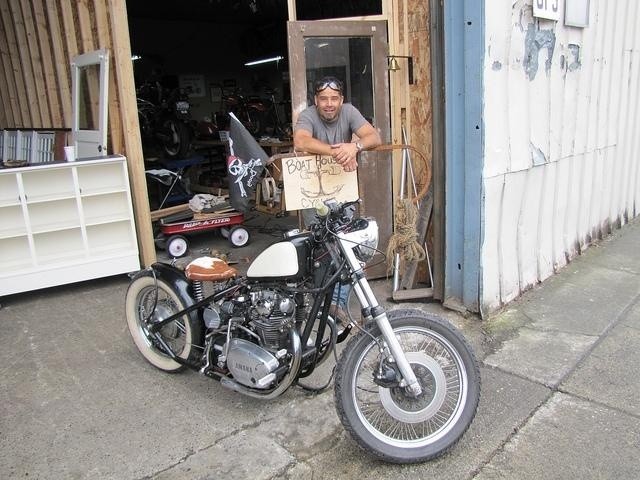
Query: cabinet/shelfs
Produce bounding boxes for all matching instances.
[0,154,141,308]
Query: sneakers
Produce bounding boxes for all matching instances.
[328,305,346,321]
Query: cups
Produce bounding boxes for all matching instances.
[63,146,75,162]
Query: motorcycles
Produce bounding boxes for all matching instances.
[137,80,192,160]
[194,91,292,142]
[124,198,483,466]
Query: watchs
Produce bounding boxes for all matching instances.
[356,141,363,152]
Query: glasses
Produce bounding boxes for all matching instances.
[317,80,341,91]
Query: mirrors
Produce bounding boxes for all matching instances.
[70,48,109,142]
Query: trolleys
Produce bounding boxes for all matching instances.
[151,192,251,258]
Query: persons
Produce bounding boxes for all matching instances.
[294,73,381,322]
